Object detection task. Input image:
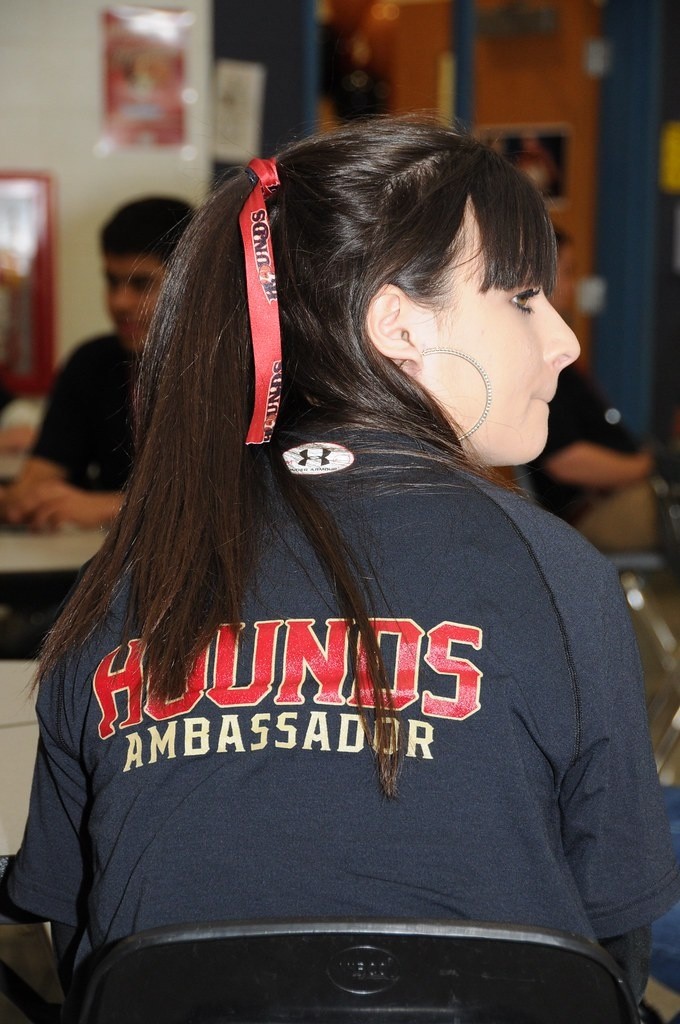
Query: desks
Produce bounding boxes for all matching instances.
[0,528,106,575]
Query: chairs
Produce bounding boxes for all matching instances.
[0,920,642,1024]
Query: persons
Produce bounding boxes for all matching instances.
[3,196,205,528]
[521,366,658,552]
[3,118,680,992]
